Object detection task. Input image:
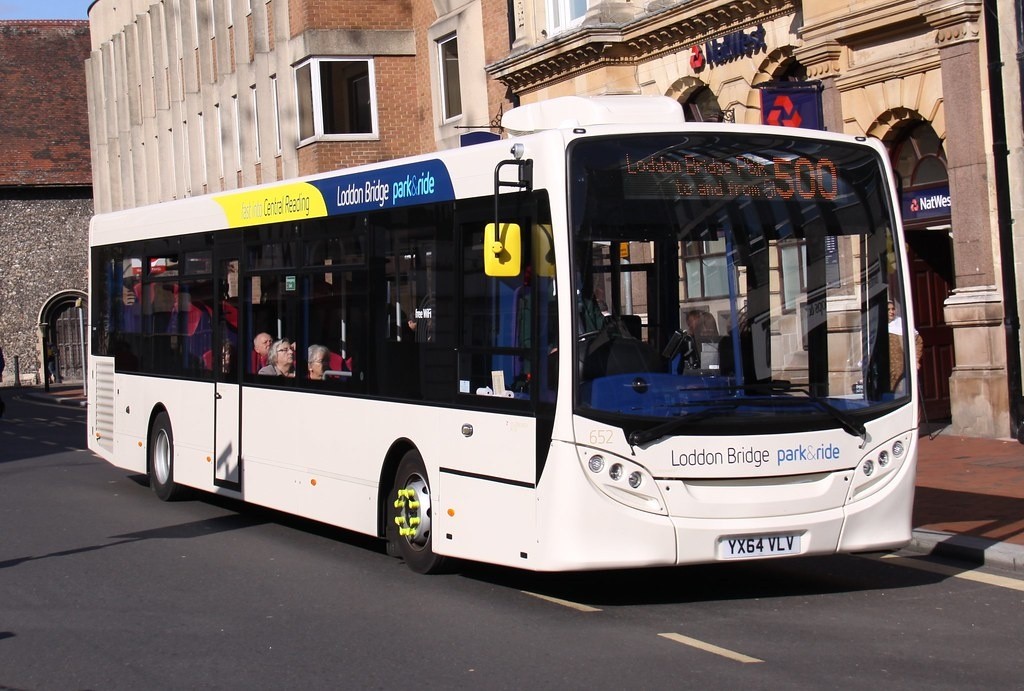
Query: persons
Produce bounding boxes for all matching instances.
[403,312,418,342]
[596,298,612,317]
[888,299,923,437]
[220,332,333,380]
[686,311,719,339]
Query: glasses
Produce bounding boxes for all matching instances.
[275,347,294,351]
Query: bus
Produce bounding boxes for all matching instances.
[83,90,921,579]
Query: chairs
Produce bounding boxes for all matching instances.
[603,316,641,340]
